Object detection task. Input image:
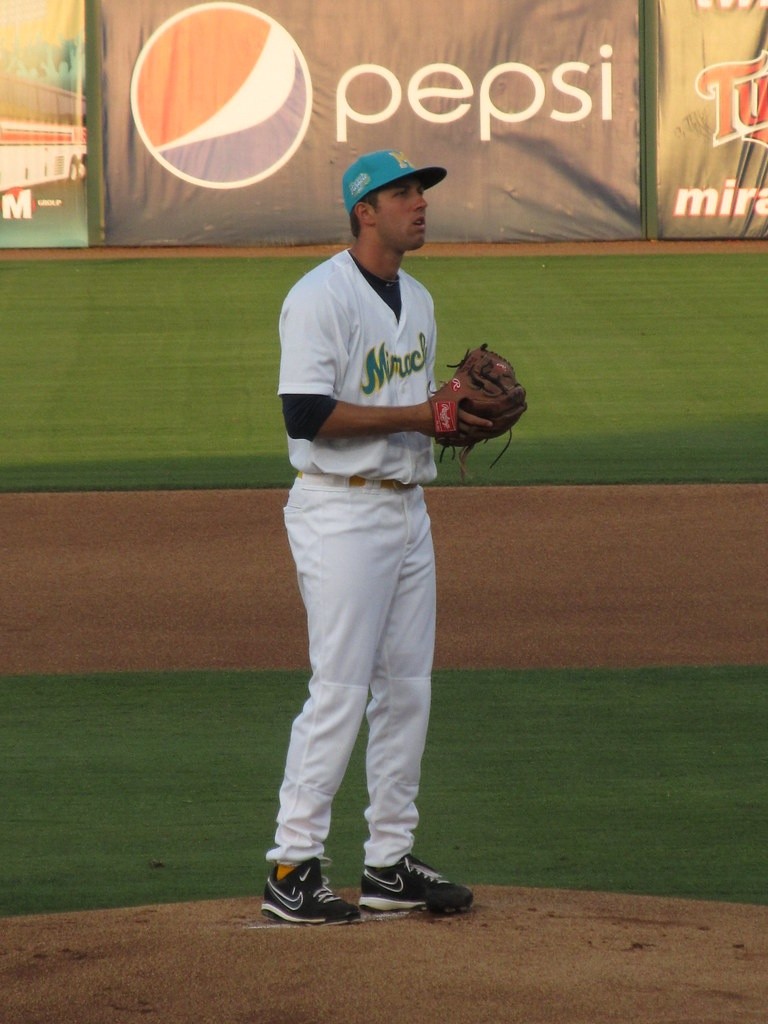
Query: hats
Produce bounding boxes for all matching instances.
[342,150,447,215]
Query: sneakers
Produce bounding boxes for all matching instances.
[261,857,360,926]
[359,853,473,913]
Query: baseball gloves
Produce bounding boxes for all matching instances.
[429,346,529,449]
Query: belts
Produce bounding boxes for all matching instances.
[298,472,417,490]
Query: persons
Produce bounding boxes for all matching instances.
[261,150,528,927]
